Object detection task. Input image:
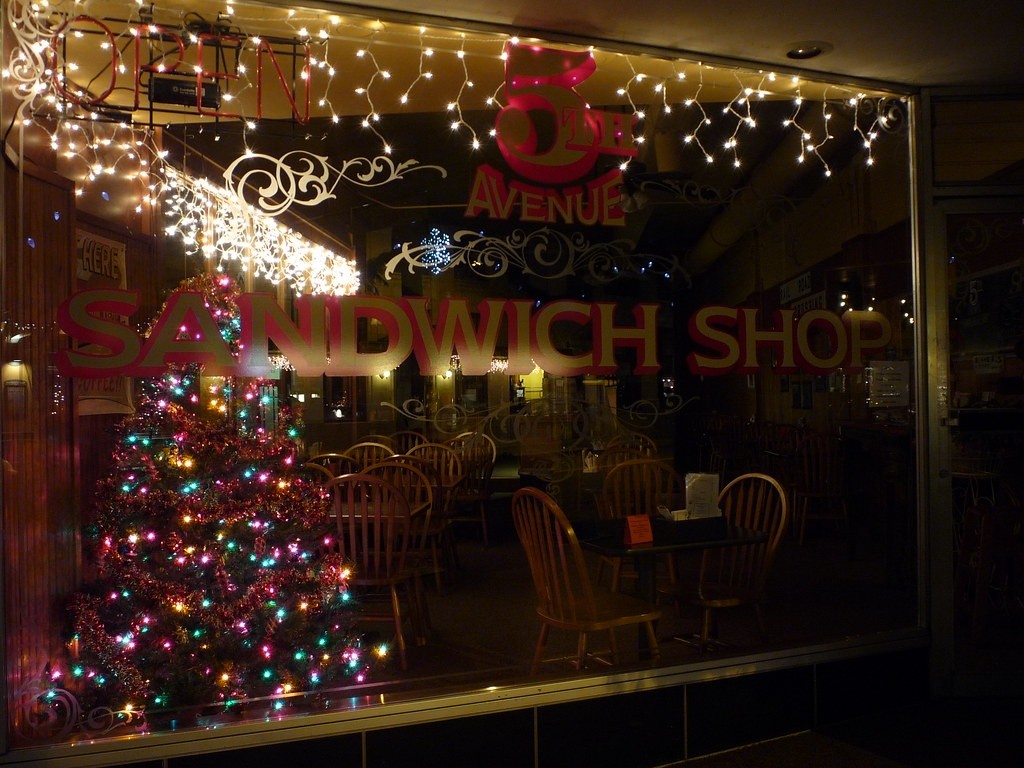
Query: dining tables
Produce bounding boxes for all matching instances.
[314,500,439,608]
[353,470,466,570]
[569,509,768,662]
[519,449,648,530]
[764,448,840,516]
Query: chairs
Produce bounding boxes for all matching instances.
[276,398,1024,672]
